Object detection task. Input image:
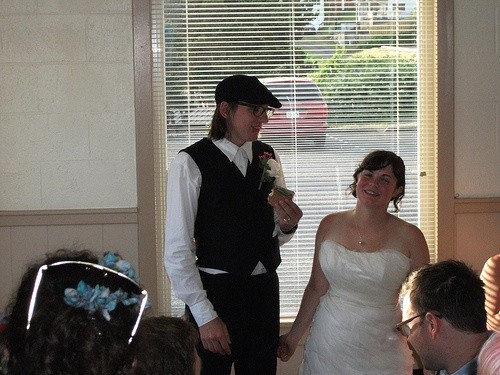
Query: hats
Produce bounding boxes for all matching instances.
[214,74,282,109]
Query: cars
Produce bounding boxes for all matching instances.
[257,77,329,149]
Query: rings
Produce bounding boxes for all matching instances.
[283,218,292,222]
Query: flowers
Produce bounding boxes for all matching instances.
[258,152,282,189]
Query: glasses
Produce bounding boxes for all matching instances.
[396,310,443,337]
[230,99,275,120]
[24,259,150,349]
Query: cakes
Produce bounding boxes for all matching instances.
[268,186,295,208]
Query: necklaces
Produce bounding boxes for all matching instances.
[357,229,368,245]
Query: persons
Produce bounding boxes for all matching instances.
[163,75,304,375]
[276,149,431,375]
[395,258,500,375]
[136,315,204,375]
[480,252,500,333]
[0,243,150,375]
